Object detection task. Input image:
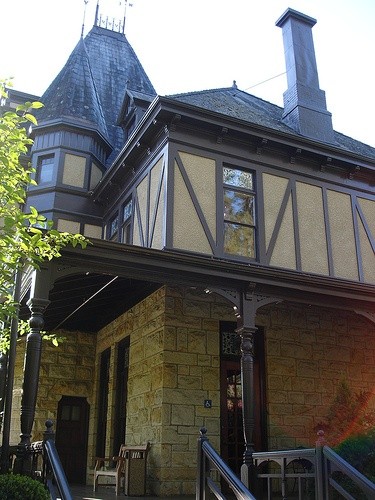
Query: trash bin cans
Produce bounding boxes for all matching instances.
[124,451,147,496]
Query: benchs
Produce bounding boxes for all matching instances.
[90,442,150,496]
